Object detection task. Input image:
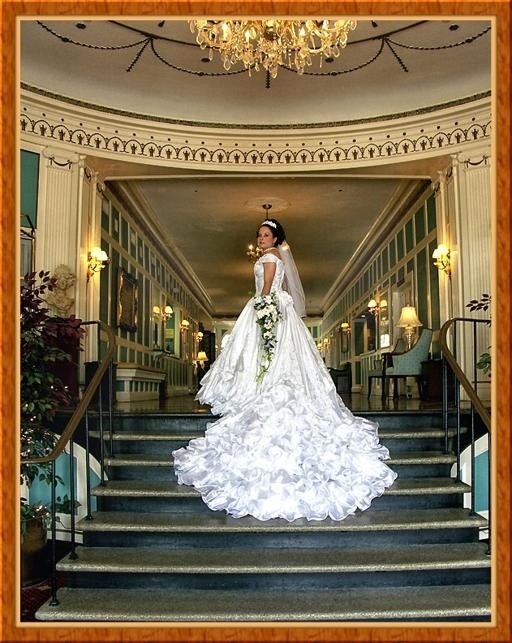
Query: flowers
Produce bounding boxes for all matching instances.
[251,288,287,388]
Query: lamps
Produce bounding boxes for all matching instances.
[183,16,363,80]
[242,202,276,267]
[86,246,113,285]
[394,303,424,352]
[315,289,380,355]
[155,304,210,371]
[428,242,453,283]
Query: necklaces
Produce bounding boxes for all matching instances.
[264,246,275,252]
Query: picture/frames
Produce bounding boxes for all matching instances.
[115,264,139,334]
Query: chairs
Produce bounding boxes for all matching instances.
[326,359,354,398]
[379,325,436,410]
[363,334,420,405]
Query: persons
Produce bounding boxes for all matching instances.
[173,217,398,521]
[48,264,75,309]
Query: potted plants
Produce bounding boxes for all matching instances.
[18,265,85,594]
